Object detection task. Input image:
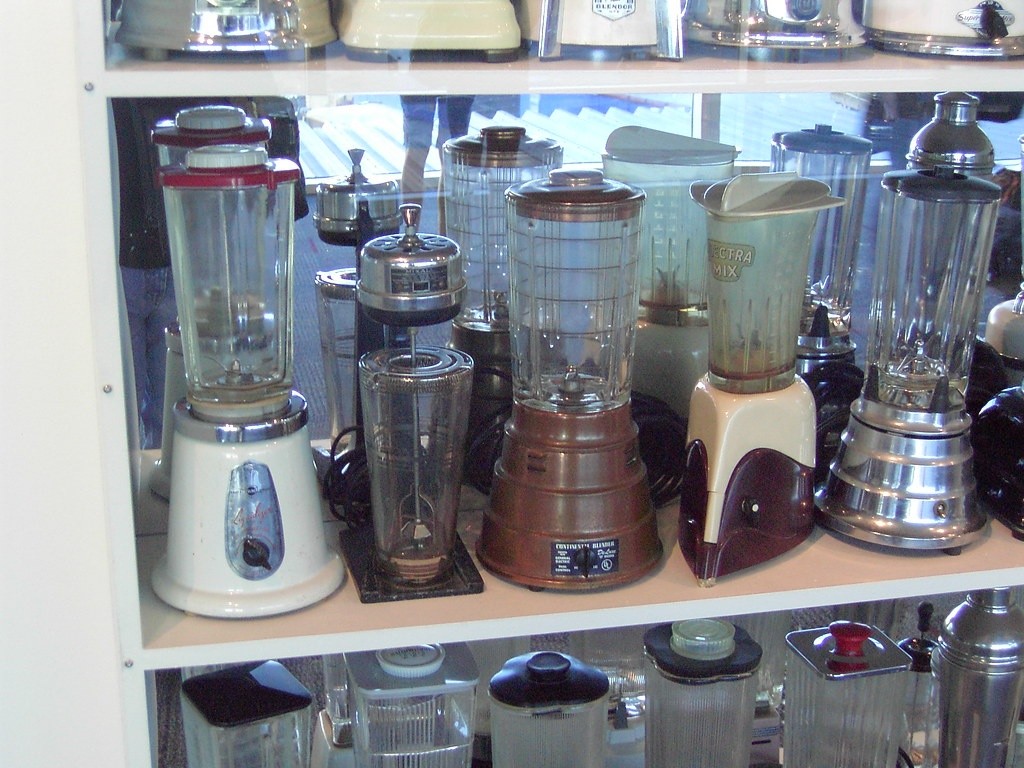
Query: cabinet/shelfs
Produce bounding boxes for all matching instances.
[72,0,1024,768]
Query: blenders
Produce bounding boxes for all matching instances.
[105,1,1024,768]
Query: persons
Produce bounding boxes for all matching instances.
[866,91,928,164]
[399,50,487,237]
